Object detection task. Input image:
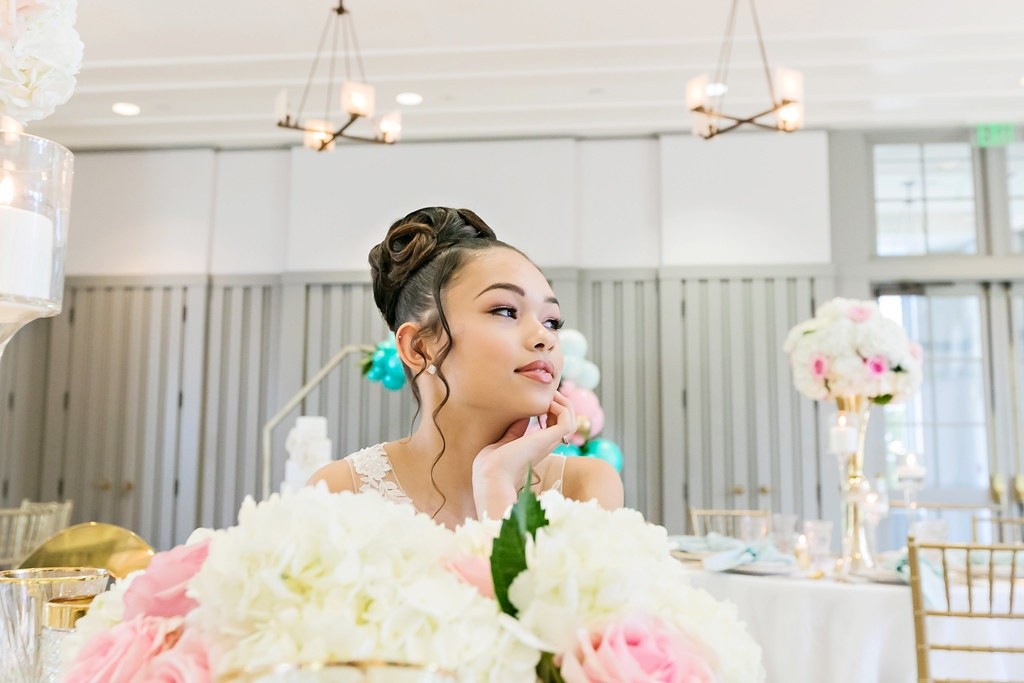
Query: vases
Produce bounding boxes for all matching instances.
[826,417,877,577]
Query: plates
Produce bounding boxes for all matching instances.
[729,553,796,574]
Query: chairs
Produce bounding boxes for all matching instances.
[689,507,772,543]
[905,535,1024,683]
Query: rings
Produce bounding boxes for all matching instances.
[562,436,569,444]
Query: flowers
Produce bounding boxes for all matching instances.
[61,460,768,683]
[780,296,926,414]
[0,0,89,123]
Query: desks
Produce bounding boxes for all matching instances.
[684,546,1024,683]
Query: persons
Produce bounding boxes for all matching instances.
[302,207,622,529]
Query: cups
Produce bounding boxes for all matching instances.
[770,514,797,551]
[805,520,833,566]
[0,567,110,683]
[42,595,96,680]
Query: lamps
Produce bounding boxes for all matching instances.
[268,0,407,154]
[685,0,810,139]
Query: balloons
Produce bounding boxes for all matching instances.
[365,335,408,390]
[523,328,624,472]
